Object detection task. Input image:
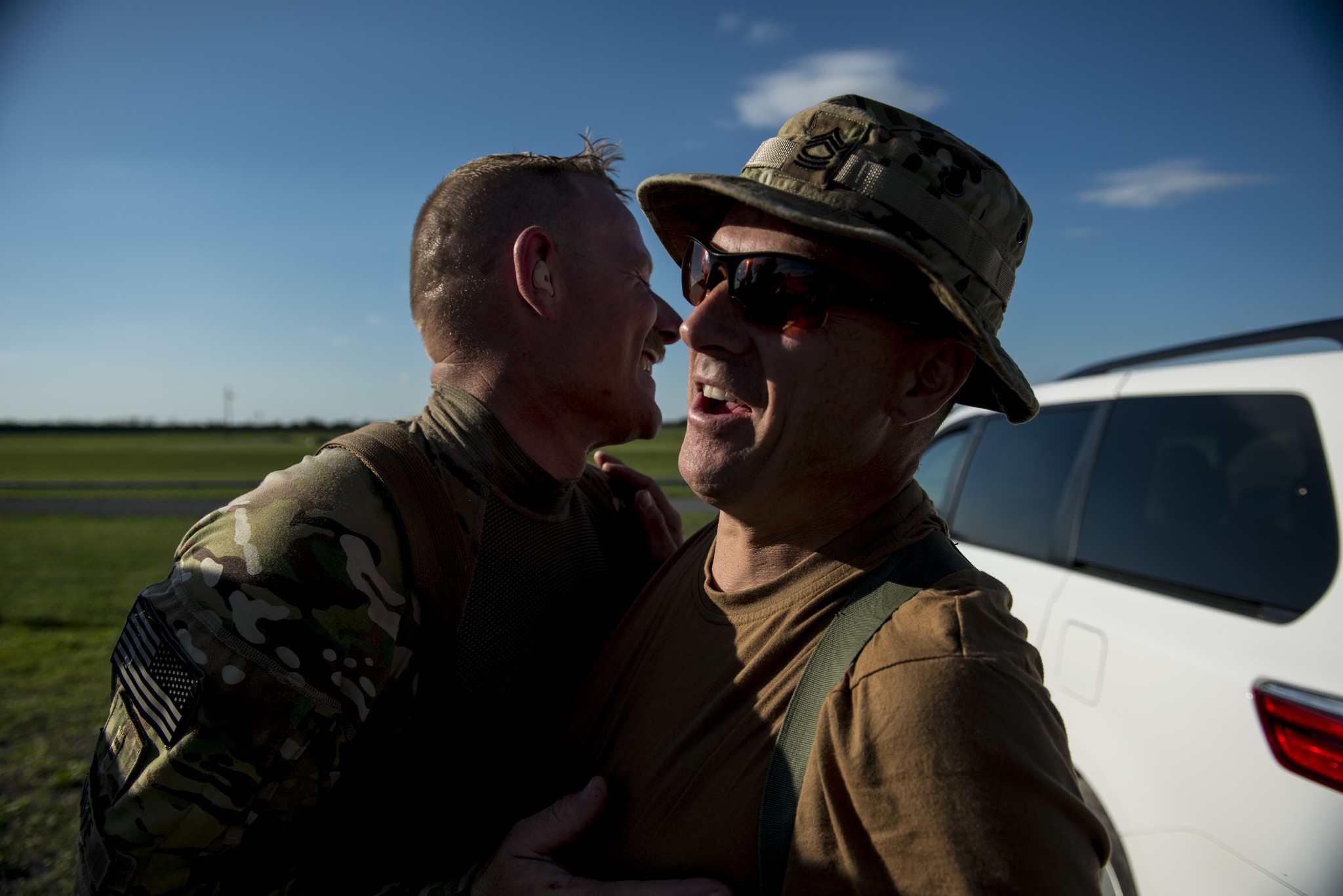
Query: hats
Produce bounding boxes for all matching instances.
[634,94,1040,425]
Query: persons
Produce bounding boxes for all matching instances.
[75,128,727,896]
[577,93,1109,896]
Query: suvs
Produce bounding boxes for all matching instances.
[915,309,1343,896]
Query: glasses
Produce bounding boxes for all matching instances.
[680,233,903,336]
